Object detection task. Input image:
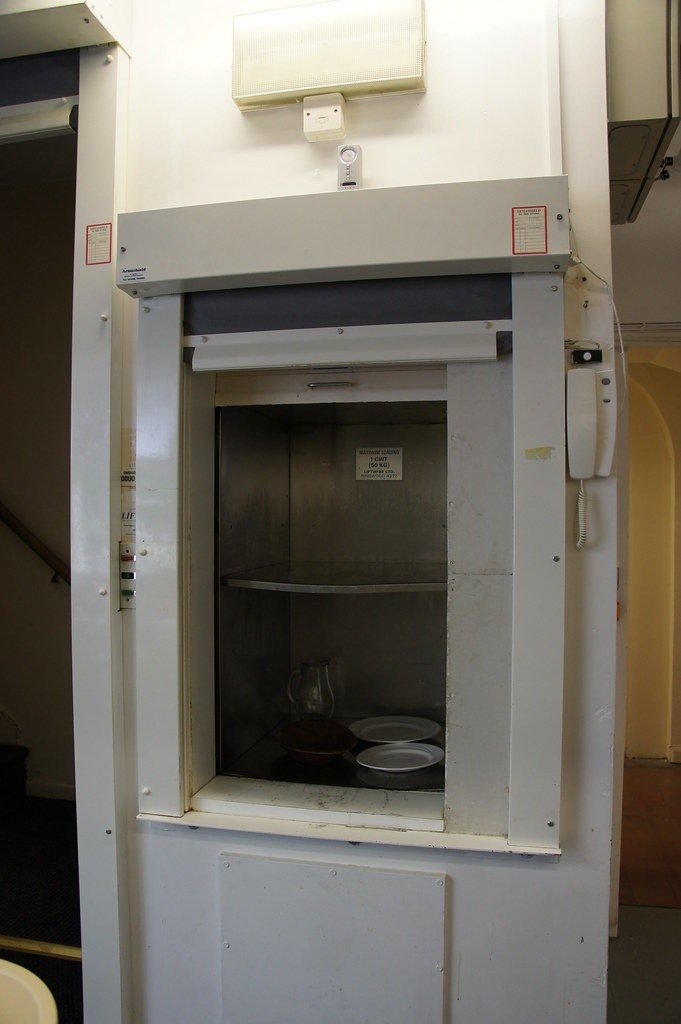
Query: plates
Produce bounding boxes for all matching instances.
[356,744,444,773]
[349,716,441,743]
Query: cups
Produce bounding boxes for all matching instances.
[286,660,335,721]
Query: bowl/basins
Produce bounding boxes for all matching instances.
[282,722,358,765]
